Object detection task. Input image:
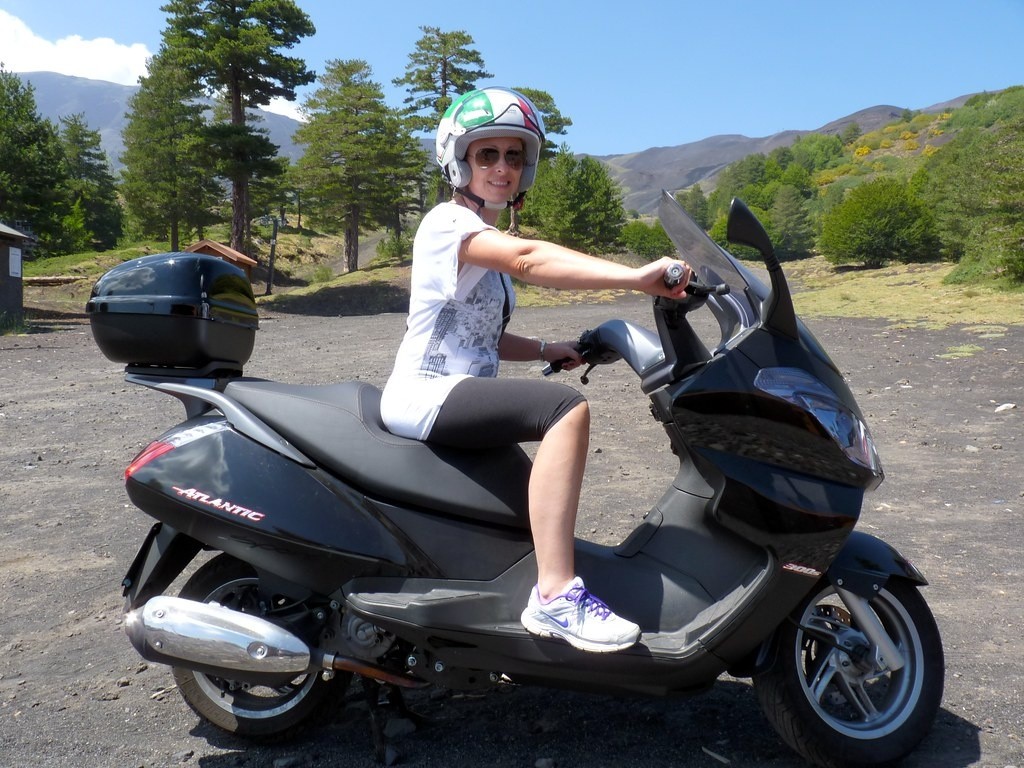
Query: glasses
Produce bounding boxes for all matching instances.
[465,145,527,171]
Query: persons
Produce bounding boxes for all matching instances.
[379,85,692,654]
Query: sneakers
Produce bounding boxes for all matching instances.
[521,576,642,654]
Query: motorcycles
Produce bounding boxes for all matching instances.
[78,185,946,768]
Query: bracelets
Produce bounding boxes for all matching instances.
[539,341,546,361]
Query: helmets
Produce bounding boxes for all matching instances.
[435,86,545,195]
